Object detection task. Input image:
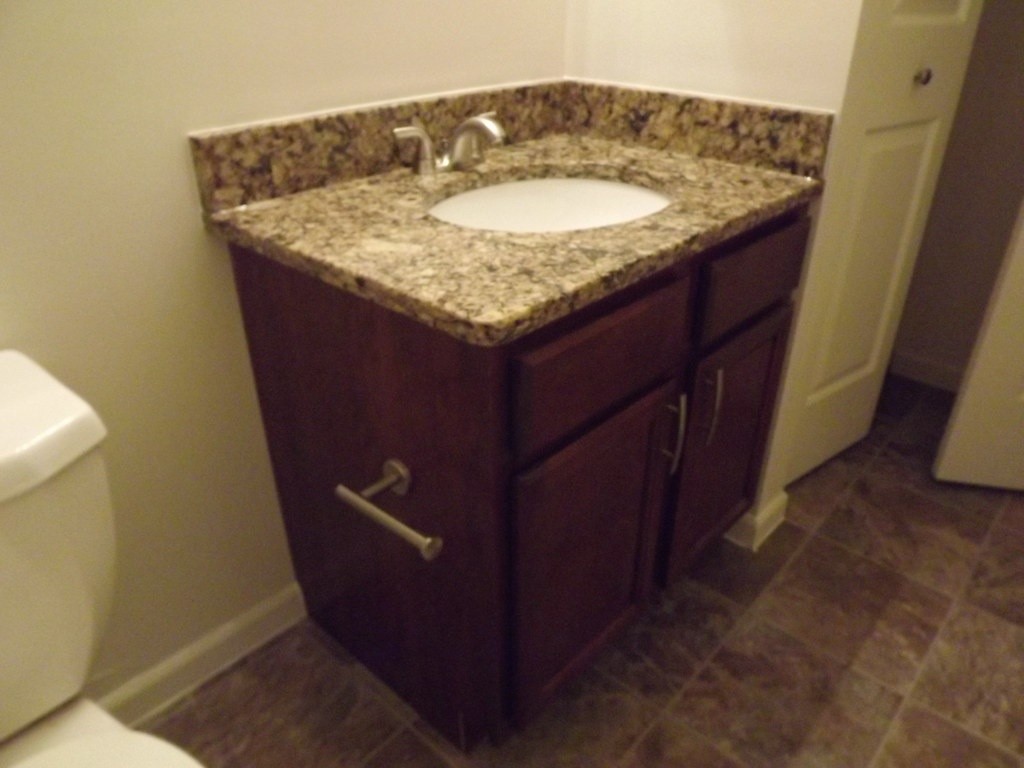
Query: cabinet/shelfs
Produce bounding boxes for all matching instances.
[210,130,826,759]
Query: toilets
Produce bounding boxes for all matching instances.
[1,344,204,767]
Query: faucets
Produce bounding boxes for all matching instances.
[444,116,504,166]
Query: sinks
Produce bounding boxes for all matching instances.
[424,158,676,236]
